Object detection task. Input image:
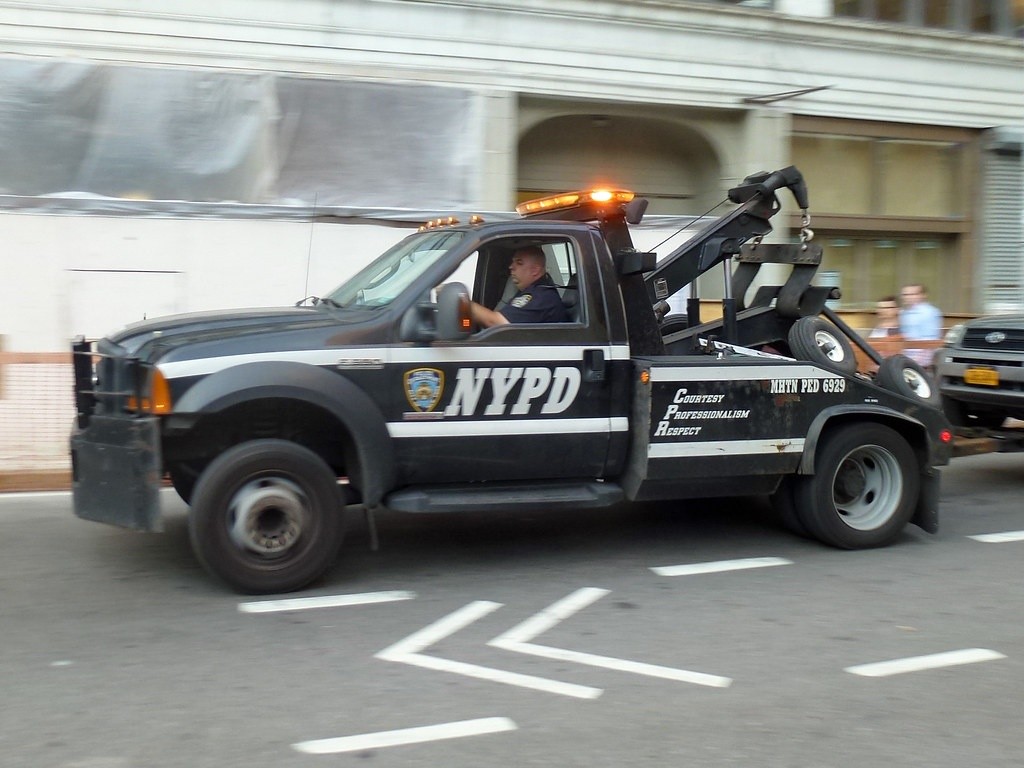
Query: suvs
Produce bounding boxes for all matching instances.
[931,315,1024,455]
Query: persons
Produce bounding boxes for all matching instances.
[867,285,940,377]
[436,247,564,328]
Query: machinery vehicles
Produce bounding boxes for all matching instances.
[64,167,958,597]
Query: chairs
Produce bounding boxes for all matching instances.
[561,273,582,323]
[492,274,518,313]
